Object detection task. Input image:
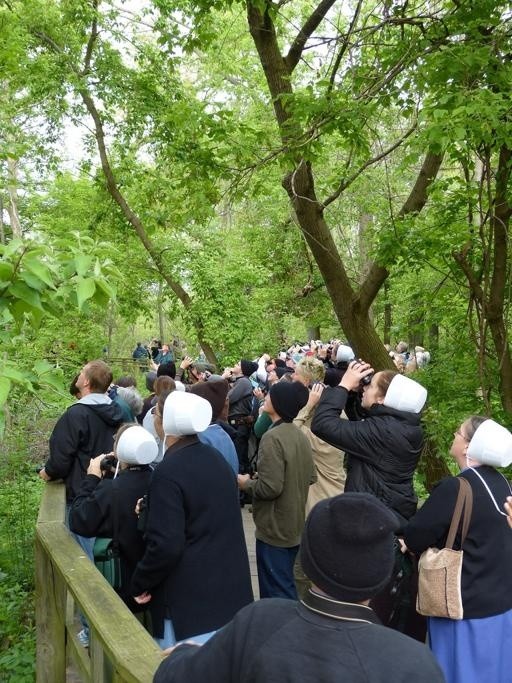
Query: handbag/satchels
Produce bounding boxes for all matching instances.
[415,474,474,621]
[92,536,122,590]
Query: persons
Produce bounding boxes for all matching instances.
[40,338,511,683]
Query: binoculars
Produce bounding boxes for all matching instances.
[259,354,273,364]
[182,356,194,368]
[347,357,373,385]
[241,467,254,479]
[100,456,117,470]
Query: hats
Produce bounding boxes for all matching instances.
[157,361,176,379]
[466,419,512,466]
[336,344,355,362]
[240,359,259,376]
[301,492,401,601]
[274,365,295,377]
[269,380,309,423]
[383,373,428,413]
[116,380,229,465]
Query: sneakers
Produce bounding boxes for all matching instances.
[77,629,89,647]
